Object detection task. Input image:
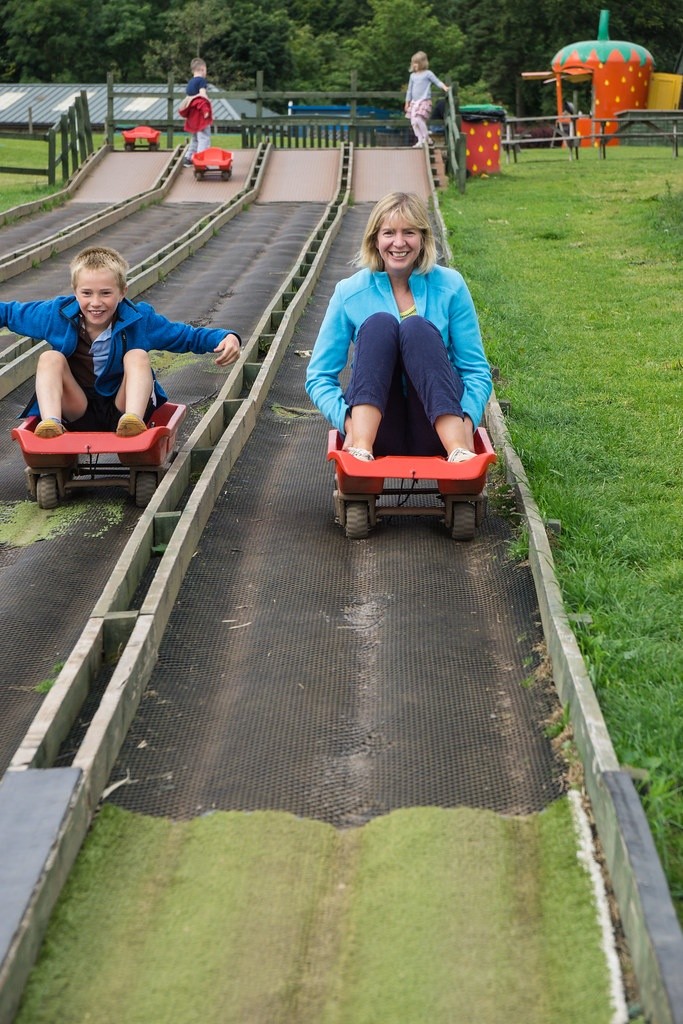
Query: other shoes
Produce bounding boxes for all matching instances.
[34,417,66,438]
[116,414,147,435]
[349,446,374,462]
[412,137,434,148]
[448,447,479,465]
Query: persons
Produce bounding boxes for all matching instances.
[179,58,213,167]
[405,51,449,149]
[305,193,492,463]
[0,247,241,438]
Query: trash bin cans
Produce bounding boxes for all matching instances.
[458,103,506,179]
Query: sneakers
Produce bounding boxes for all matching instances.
[183,157,194,167]
